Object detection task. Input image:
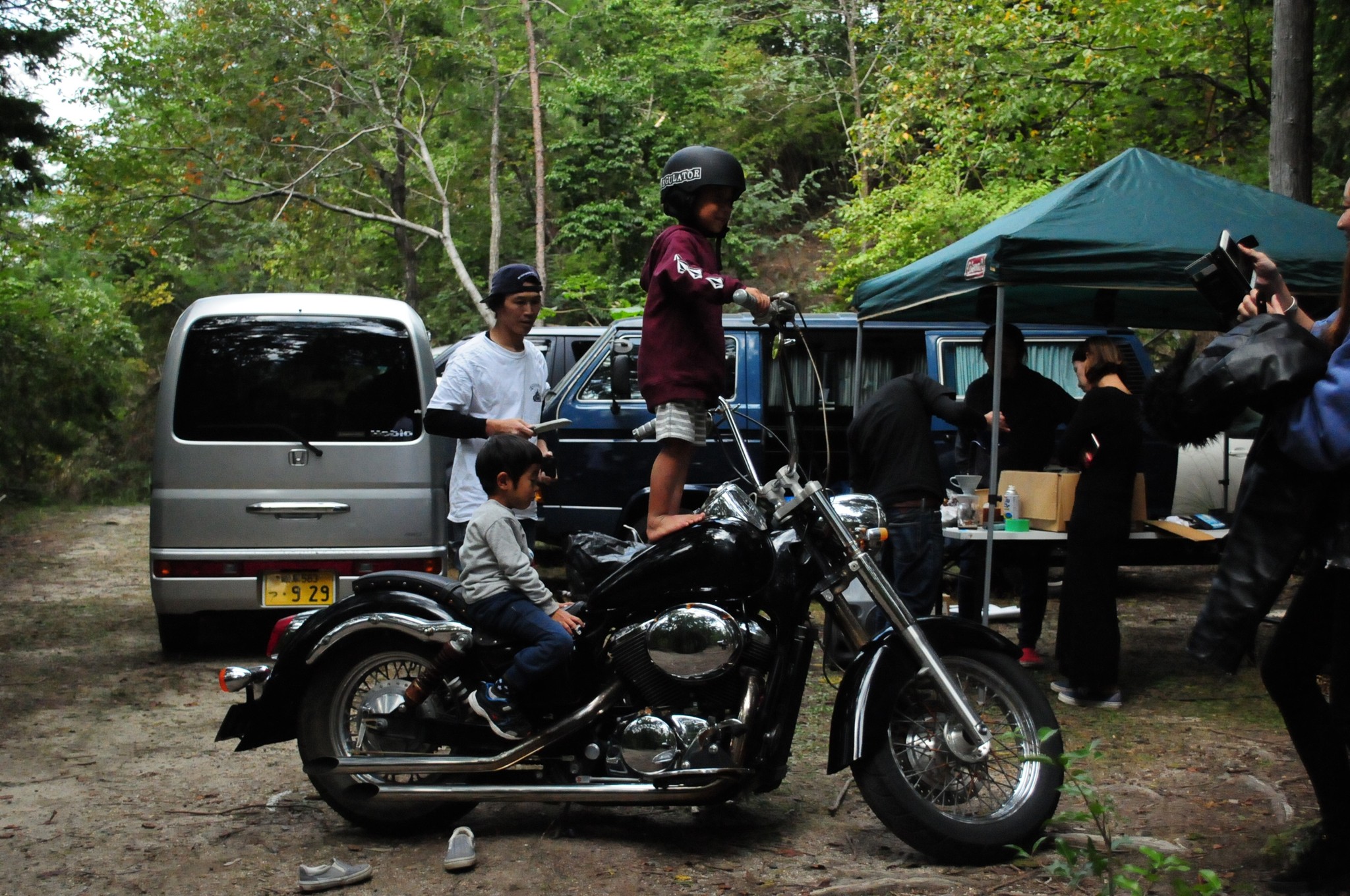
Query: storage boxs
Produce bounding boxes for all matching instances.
[998,470,1146,532]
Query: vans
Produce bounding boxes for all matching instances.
[428,326,607,422]
[149,294,451,649]
[535,308,1181,584]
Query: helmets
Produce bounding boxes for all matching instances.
[660,146,746,204]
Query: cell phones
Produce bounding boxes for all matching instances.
[1217,230,1257,291]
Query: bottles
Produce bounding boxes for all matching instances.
[955,496,980,530]
[982,502,1001,530]
[1003,486,1020,522]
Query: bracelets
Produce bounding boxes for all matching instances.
[1282,296,1298,317]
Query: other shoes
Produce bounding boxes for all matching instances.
[1058,691,1121,708]
[299,858,371,890]
[444,826,477,870]
[1020,648,1041,666]
[1051,682,1076,693]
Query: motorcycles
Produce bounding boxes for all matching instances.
[212,288,1069,865]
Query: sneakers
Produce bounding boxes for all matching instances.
[468,682,530,740]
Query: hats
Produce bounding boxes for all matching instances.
[480,264,543,303]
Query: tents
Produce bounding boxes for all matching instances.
[851,148,1350,704]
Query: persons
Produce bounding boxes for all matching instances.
[954,322,1076,668]
[1236,176,1350,896]
[722,637,728,648]
[1049,333,1139,715]
[422,263,560,574]
[637,145,770,541]
[848,368,1011,634]
[458,434,584,739]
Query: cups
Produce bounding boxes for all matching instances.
[950,475,982,496]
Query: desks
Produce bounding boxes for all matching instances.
[934,528,1229,617]
